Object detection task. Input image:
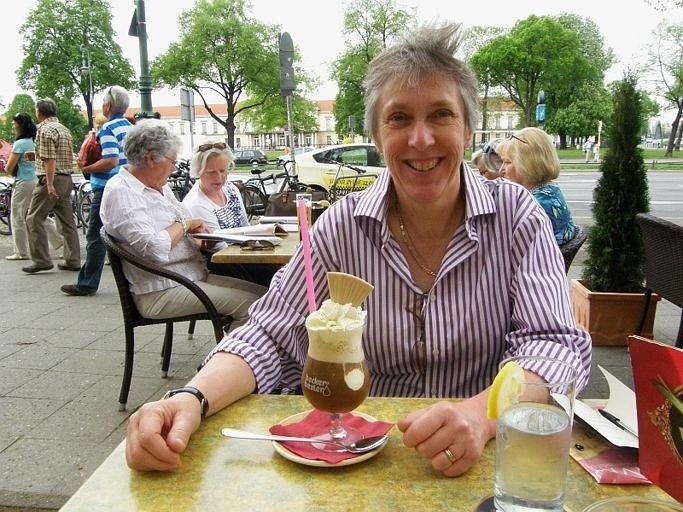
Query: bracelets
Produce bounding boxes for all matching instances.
[174,216,189,238]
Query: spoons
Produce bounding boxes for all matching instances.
[221,428,388,453]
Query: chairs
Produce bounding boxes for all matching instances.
[99,224,224,413]
[559,224,587,275]
[633,213,683,351]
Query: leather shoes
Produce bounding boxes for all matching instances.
[58,263,81,271]
[61,285,83,296]
[22,265,53,273]
[6,254,29,260]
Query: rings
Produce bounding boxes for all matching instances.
[444,448,457,465]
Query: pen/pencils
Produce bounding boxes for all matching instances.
[598,407,639,441]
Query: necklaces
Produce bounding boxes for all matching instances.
[393,200,448,277]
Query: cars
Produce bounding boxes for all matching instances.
[276,146,315,167]
[285,144,386,202]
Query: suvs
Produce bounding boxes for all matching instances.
[233,149,267,164]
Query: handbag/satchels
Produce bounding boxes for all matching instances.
[266,183,328,217]
[78,130,101,167]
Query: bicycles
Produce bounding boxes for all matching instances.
[0,181,12,236]
[315,155,366,203]
[238,158,308,225]
[70,180,95,228]
[179,160,253,223]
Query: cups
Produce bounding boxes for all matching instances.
[296,194,312,243]
[494,356,578,512]
[301,314,370,453]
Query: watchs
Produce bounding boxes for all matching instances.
[161,387,210,421]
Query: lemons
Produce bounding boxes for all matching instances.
[486,361,526,418]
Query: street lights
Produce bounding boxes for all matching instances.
[79,45,95,129]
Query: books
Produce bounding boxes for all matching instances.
[188,219,287,248]
[258,210,299,232]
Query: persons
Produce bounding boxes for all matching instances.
[126,24,594,480]
[181,136,250,253]
[59,86,135,296]
[99,117,268,333]
[498,127,575,248]
[471,137,506,182]
[21,99,81,274]
[4,111,64,261]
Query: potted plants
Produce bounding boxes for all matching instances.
[570,78,661,347]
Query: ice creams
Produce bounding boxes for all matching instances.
[301,269,375,413]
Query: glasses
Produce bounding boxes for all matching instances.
[108,86,115,103]
[508,134,527,145]
[405,292,427,373]
[481,142,497,156]
[241,240,274,251]
[163,154,178,165]
[15,112,23,119]
[197,143,226,153]
